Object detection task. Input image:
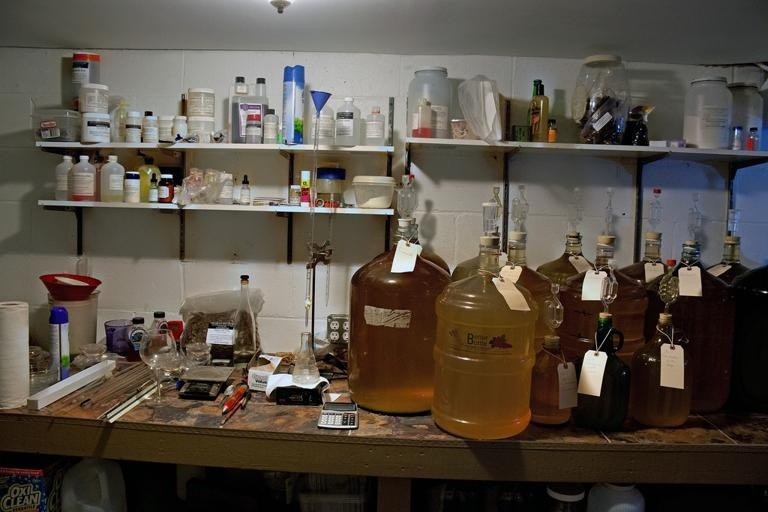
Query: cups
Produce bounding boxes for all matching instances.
[104,318,134,357]
[186,341,212,367]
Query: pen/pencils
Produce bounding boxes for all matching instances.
[220,380,251,426]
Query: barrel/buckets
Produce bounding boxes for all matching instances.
[726,82,764,150]
[49,291,100,354]
[684,77,731,148]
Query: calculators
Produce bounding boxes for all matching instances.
[317,402,359,430]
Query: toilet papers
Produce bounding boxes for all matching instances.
[0,301,29,409]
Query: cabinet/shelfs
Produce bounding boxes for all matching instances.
[33,136,398,222]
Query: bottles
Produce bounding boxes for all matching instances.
[346,182,768,441]
[28,344,56,396]
[127,310,167,360]
[294,330,320,384]
[289,184,300,206]
[682,76,764,149]
[571,54,631,146]
[315,166,346,208]
[546,118,557,143]
[450,118,476,141]
[53,150,175,203]
[300,170,310,208]
[36,52,387,146]
[528,81,549,142]
[232,274,256,366]
[239,174,250,205]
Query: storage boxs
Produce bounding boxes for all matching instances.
[350,174,399,212]
[31,107,82,145]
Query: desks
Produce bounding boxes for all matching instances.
[0,343,768,512]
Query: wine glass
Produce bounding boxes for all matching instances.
[139,325,173,408]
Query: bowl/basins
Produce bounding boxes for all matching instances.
[38,272,102,300]
[352,175,396,209]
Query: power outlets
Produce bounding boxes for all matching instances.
[326,312,350,345]
